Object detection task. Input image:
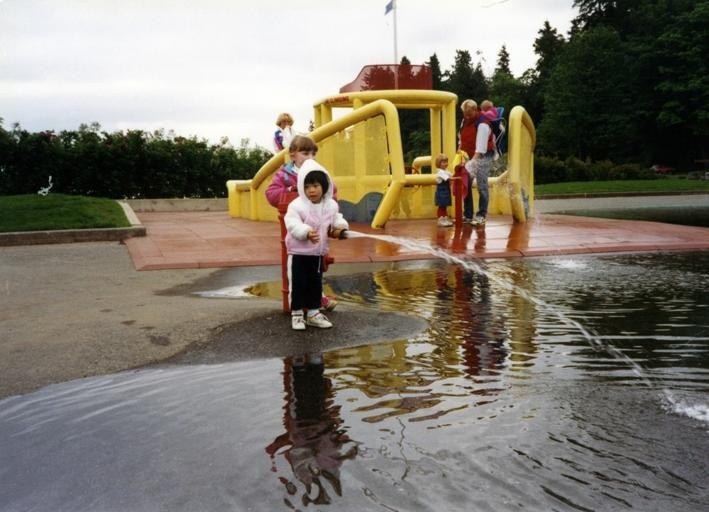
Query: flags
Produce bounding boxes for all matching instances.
[383,0,396,16]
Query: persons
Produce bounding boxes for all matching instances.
[435,154,463,227]
[283,159,352,331]
[457,99,506,227]
[274,112,299,151]
[266,136,337,315]
[479,99,499,162]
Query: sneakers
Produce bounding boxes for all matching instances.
[306,313,334,328]
[292,311,306,330]
[437,216,485,227]
